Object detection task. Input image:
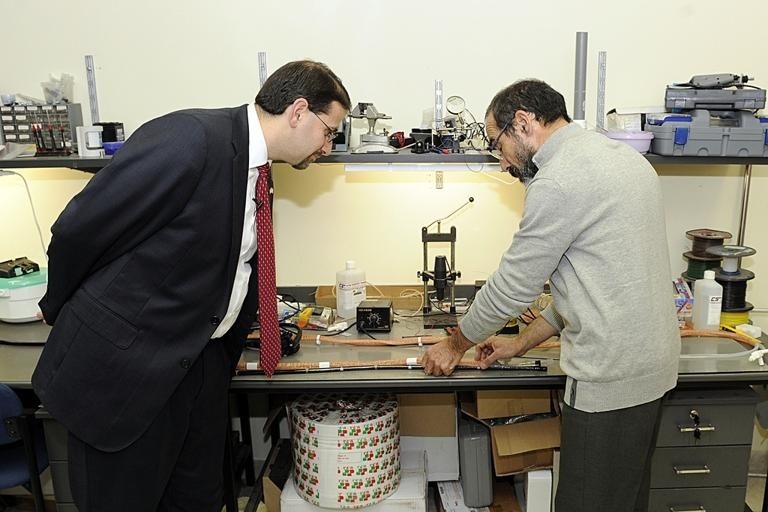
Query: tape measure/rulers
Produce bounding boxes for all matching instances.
[236,365,548,377]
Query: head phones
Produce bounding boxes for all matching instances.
[276,322,302,358]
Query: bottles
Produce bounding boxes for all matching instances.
[337,261,366,317]
[692,269,724,333]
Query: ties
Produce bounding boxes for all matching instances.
[256,165,283,376]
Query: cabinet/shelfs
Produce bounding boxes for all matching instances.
[0,147,768,512]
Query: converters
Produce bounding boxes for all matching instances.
[355,300,395,332]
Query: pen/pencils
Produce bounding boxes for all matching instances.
[402,334,433,338]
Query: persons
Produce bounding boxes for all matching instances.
[418,77,684,510]
[29,57,352,511]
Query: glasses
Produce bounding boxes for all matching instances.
[314,111,340,144]
[487,117,515,161]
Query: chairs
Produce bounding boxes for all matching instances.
[0,382,53,512]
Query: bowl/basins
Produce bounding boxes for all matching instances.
[608,131,654,158]
